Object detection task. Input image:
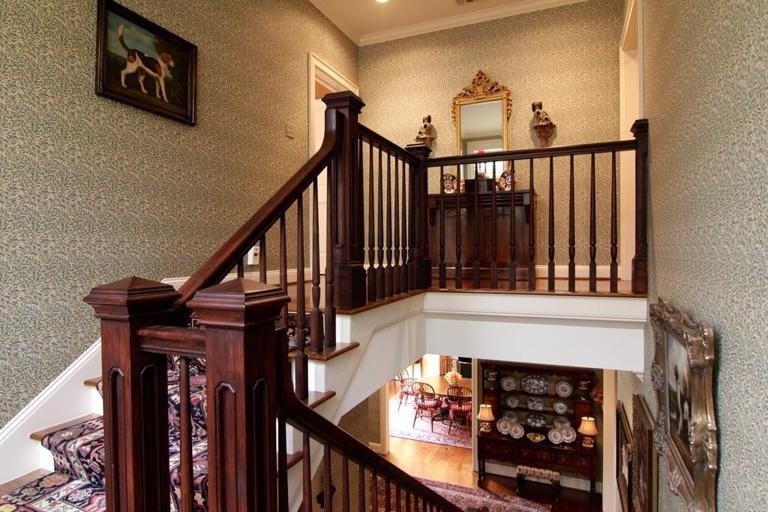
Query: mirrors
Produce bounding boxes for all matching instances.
[451,71,513,183]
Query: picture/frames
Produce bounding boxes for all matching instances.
[95,0,198,127]
[616,297,720,512]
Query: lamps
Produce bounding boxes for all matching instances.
[477,401,495,433]
[577,415,599,448]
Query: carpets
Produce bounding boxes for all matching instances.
[369,470,553,512]
[389,386,472,450]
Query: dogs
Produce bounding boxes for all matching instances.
[116,22,178,103]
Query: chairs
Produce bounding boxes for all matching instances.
[396,368,472,435]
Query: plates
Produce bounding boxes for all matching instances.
[552,399,568,415]
[554,381,574,398]
[496,410,577,445]
[499,169,517,191]
[500,375,517,392]
[520,373,550,395]
[505,394,520,409]
[526,395,545,412]
[439,173,458,193]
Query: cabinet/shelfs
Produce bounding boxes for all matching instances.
[478,360,602,504]
